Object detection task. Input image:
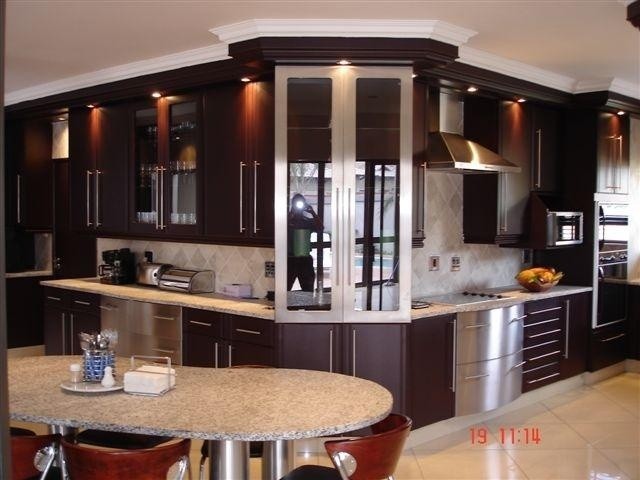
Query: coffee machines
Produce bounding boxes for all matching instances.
[98,249,135,285]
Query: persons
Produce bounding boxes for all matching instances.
[288,194,324,293]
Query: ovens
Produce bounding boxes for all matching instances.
[592,201,632,331]
[547,210,585,247]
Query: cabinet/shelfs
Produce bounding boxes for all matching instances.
[202,80,275,250]
[5,119,53,232]
[564,106,630,195]
[461,90,531,245]
[412,75,429,247]
[126,90,202,244]
[40,284,102,355]
[182,306,280,368]
[593,321,628,373]
[276,320,406,426]
[405,312,457,429]
[275,66,411,321]
[68,101,128,240]
[531,98,564,190]
[523,290,592,394]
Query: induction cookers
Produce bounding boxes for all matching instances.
[418,290,513,307]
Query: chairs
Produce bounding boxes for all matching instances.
[9,426,70,479]
[275,413,413,479]
[197,364,277,480]
[60,429,193,479]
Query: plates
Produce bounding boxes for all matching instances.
[60,377,126,394]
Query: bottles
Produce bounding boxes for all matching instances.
[100,365,116,387]
[67,364,84,383]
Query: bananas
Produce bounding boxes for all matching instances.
[514,268,562,284]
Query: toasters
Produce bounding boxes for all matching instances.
[135,261,173,285]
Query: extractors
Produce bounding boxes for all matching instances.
[413,75,522,174]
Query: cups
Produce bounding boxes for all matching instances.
[135,210,157,225]
[169,213,196,225]
[170,160,197,171]
[144,117,199,136]
